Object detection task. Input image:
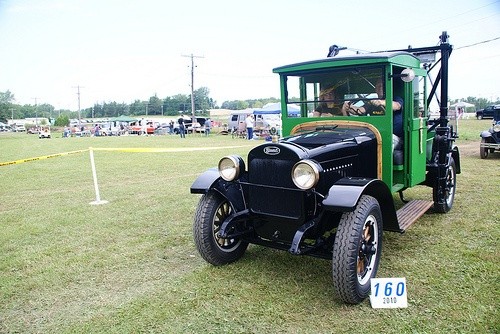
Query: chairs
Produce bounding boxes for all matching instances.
[393,149,404,166]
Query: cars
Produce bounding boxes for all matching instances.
[5,102,311,136]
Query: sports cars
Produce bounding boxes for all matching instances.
[479,117,500,160]
[189,28,462,305]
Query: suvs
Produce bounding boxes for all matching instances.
[474,103,500,120]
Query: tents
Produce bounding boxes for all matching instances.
[107,115,138,134]
[450,102,476,120]
[253,102,301,139]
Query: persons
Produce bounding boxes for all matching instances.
[204,117,211,136]
[140,117,148,136]
[246,113,254,141]
[344,79,403,148]
[167,120,174,134]
[69,124,107,137]
[309,85,350,116]
[178,115,186,138]
